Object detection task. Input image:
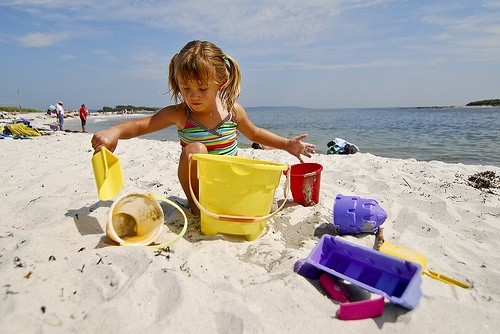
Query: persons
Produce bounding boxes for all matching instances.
[79,103,88,133]
[90,40,317,216]
[1,102,135,120]
[57,101,64,131]
[250,141,278,150]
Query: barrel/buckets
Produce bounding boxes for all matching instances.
[105,192,187,248]
[187,153,291,241]
[283,163,323,206]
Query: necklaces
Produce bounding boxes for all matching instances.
[207,112,215,117]
